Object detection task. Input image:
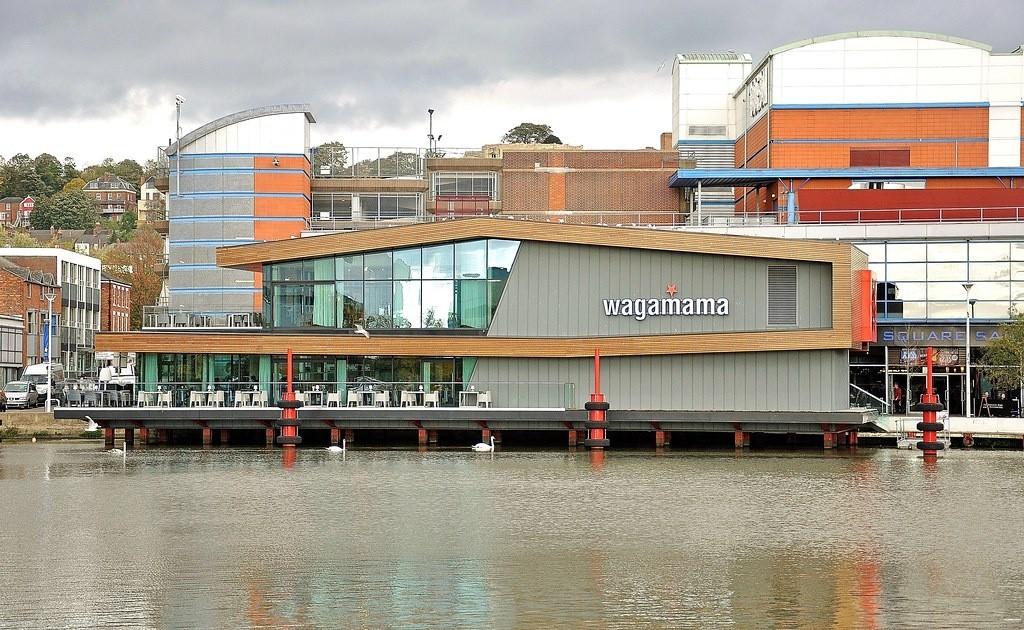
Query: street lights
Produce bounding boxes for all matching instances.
[44,293,57,411]
[962,284,974,419]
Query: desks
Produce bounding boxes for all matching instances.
[82,392,111,407]
[356,391,376,407]
[458,391,479,407]
[195,392,214,406]
[406,391,425,406]
[144,392,163,407]
[242,392,261,407]
[304,391,323,407]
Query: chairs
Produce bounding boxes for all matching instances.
[295,390,493,408]
[67,389,269,408]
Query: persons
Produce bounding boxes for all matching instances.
[214,377,222,390]
[978,390,1012,418]
[195,377,202,385]
[916,381,925,397]
[250,375,260,391]
[324,379,334,392]
[893,380,901,413]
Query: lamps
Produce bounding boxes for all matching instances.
[272,156,279,166]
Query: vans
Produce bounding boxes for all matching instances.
[20,362,63,406]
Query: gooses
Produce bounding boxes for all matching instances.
[107,441,127,453]
[324,439,346,449]
[471,436,495,448]
[85,416,101,432]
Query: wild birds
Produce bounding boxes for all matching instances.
[354,323,371,339]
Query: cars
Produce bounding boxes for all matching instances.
[2,381,39,410]
